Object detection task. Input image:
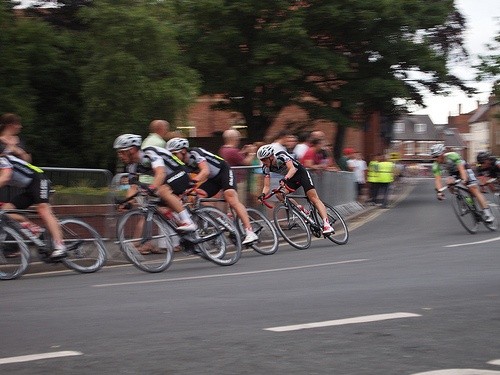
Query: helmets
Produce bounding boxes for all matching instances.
[476,152,490,163]
[166,137,190,151]
[113,133,142,150]
[430,144,446,155]
[257,145,276,159]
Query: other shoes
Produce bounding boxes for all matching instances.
[27,223,46,244]
[485,215,495,223]
[141,244,152,254]
[43,246,69,263]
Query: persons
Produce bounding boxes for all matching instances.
[256,143,335,234]
[431,143,495,222]
[476,151,500,191]
[347,151,398,206]
[141,120,173,152]
[166,137,259,244]
[113,134,198,254]
[219,129,259,206]
[0,113,67,260]
[250,131,354,171]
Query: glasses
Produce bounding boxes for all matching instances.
[171,149,184,156]
[478,158,487,164]
[431,152,444,159]
[260,157,270,161]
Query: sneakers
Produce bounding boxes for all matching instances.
[176,217,197,233]
[298,205,310,223]
[322,217,334,233]
[241,228,259,245]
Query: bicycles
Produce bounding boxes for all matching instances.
[114,189,280,273]
[437,178,497,235]
[260,184,349,251]
[0,207,107,281]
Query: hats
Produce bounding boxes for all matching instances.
[223,129,245,139]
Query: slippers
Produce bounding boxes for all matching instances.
[154,245,183,253]
